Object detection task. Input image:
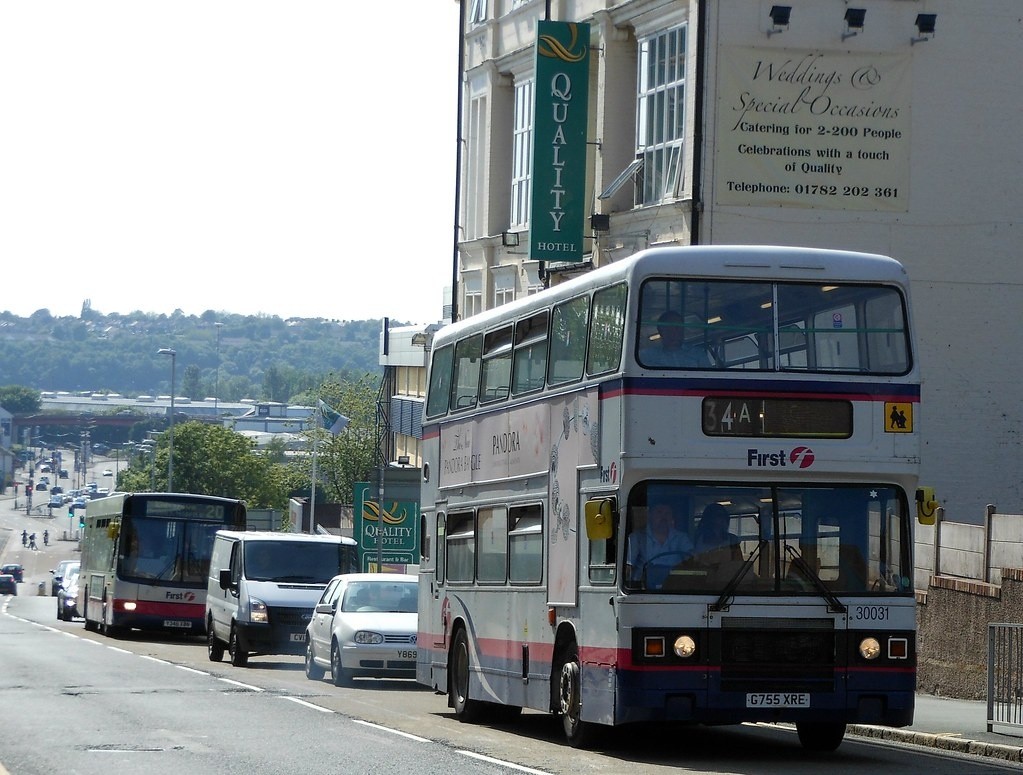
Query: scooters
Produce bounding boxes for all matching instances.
[21,534,48,550]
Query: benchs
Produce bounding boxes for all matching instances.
[455,374,580,409]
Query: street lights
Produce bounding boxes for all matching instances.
[156,347,178,492]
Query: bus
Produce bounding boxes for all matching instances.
[414,242,938,754]
[76,492,257,639]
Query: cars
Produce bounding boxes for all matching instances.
[48,560,81,623]
[305,572,420,688]
[135,438,156,456]
[20,451,113,508]
[0,574,18,596]
[0,564,25,583]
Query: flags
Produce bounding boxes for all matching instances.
[317,398,349,435]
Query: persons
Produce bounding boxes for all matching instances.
[26,533,39,549]
[695,503,744,569]
[22,530,28,544]
[627,496,697,589]
[646,310,714,368]
[43,529,48,543]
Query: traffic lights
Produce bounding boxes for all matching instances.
[79,516,85,529]
[69,507,74,518]
[14,483,18,486]
[28,486,33,490]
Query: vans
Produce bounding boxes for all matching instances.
[204,529,358,667]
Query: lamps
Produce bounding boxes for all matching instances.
[766,5,793,40]
[501,232,520,248]
[910,13,938,46]
[591,213,610,233]
[841,8,867,43]
[398,455,409,468]
